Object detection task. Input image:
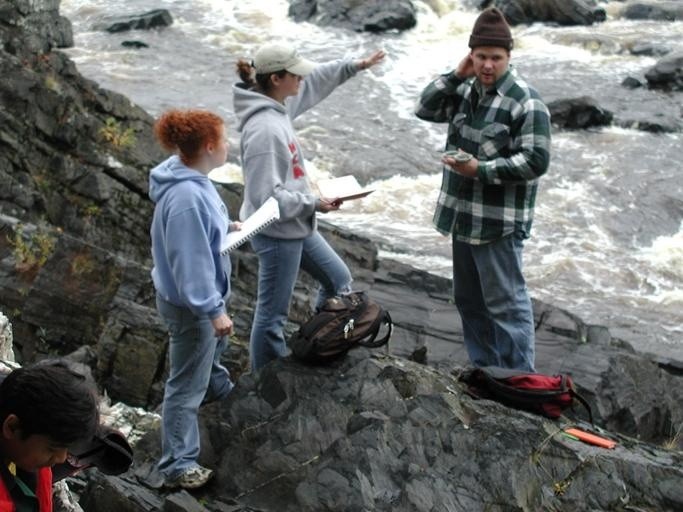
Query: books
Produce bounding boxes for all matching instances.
[221,197,279,256]
[315,174,375,203]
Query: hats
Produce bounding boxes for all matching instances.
[52,426,133,483]
[251,41,316,77]
[468,8,513,50]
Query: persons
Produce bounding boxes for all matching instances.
[149,111,233,491]
[414,9,551,371]
[233,42,386,366]
[0,362,98,512]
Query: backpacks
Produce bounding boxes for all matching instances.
[457,360,594,427]
[291,291,394,365]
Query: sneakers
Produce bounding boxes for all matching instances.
[163,464,213,489]
[450,365,472,378]
